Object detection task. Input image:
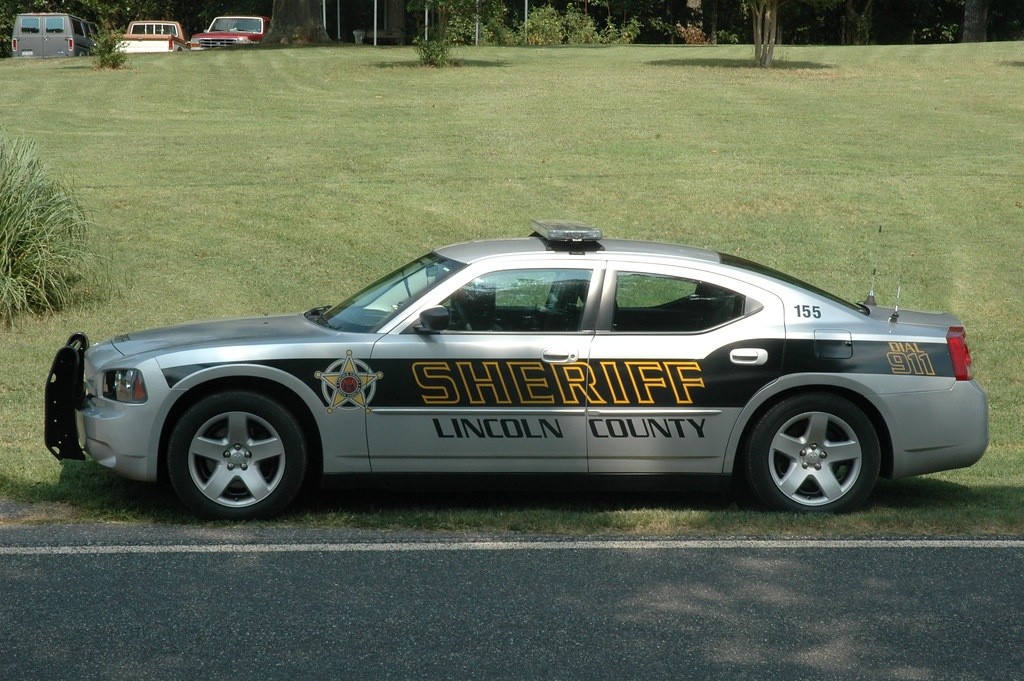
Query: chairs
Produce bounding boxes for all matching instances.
[548,271,581,330]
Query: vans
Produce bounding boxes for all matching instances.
[11,12,99,57]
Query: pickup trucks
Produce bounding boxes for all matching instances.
[114,20,190,54]
[190,16,271,52]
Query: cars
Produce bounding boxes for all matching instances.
[43,220,992,513]
